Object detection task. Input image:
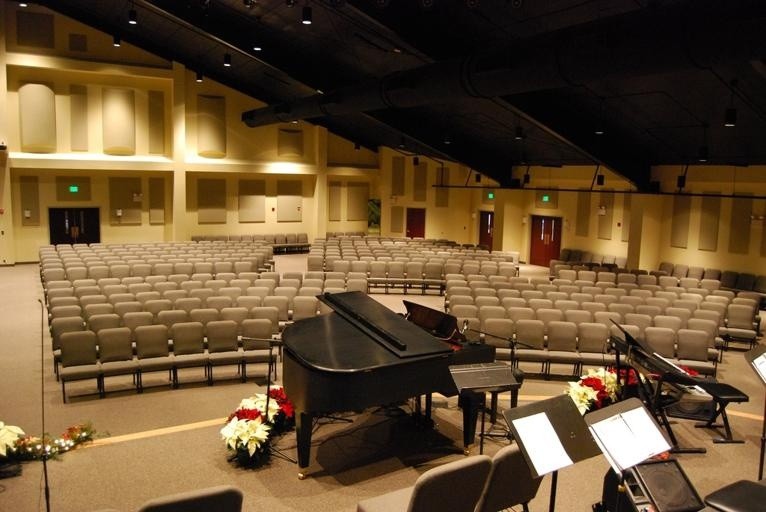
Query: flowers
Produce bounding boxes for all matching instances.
[566,368,620,416]
[220,385,296,459]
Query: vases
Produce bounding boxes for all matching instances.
[238,448,273,470]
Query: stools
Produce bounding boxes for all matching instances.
[705,480,766,511]
[697,383,747,444]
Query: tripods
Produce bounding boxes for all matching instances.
[468,328,534,445]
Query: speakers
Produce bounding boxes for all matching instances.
[597,174,605,185]
[412,156,418,164]
[474,173,480,182]
[523,173,530,182]
[676,174,685,187]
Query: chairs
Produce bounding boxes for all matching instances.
[276,234,287,252]
[392,237,546,378]
[287,234,298,252]
[475,442,544,512]
[123,274,206,393]
[298,233,311,252]
[358,455,493,512]
[39,242,151,280]
[311,231,390,294]
[547,249,656,379]
[139,486,243,512]
[44,278,123,403]
[151,234,275,274]
[206,272,323,386]
[657,262,766,378]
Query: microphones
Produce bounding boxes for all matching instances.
[462,318,471,337]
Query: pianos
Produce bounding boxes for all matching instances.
[282,291,496,481]
[609,335,714,404]
